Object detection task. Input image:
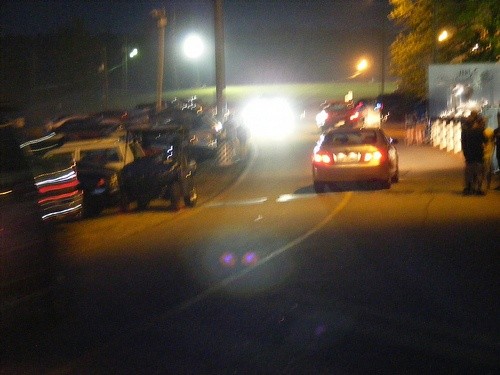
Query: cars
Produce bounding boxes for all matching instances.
[312,128,399,191]
[0,98,227,224]
[318,99,362,131]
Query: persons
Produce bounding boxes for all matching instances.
[460,109,500,196]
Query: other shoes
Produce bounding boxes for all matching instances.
[465,186,499,197]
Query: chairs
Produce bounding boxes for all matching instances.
[362,136,376,143]
[333,136,350,145]
